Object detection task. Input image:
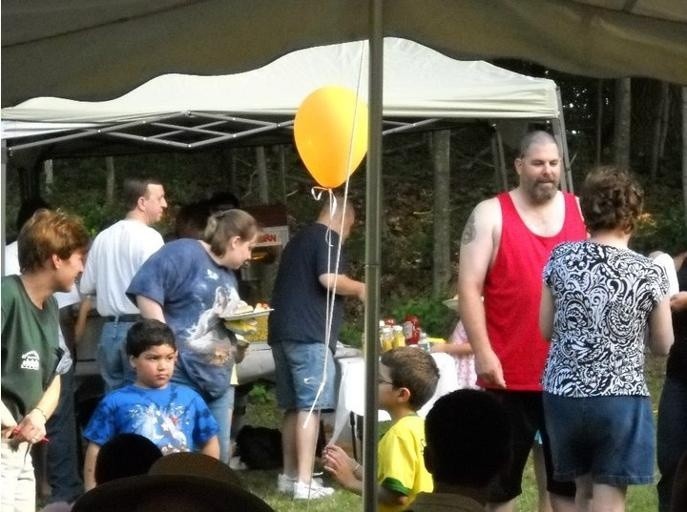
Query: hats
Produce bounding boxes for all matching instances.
[69,452,278,512]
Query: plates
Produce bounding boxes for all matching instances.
[439,297,485,310]
[215,306,277,321]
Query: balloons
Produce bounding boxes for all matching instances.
[294,84,369,191]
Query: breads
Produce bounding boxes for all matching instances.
[233,306,254,315]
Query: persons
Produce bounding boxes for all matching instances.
[456,129,590,511]
[656,249,687,512]
[539,166,675,512]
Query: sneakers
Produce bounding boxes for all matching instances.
[276,473,324,493]
[292,477,335,501]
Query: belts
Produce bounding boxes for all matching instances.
[97,314,144,322]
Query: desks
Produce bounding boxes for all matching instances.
[334,350,459,464]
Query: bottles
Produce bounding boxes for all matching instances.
[378,315,431,358]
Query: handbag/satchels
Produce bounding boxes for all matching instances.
[234,424,284,471]
[1,428,37,512]
